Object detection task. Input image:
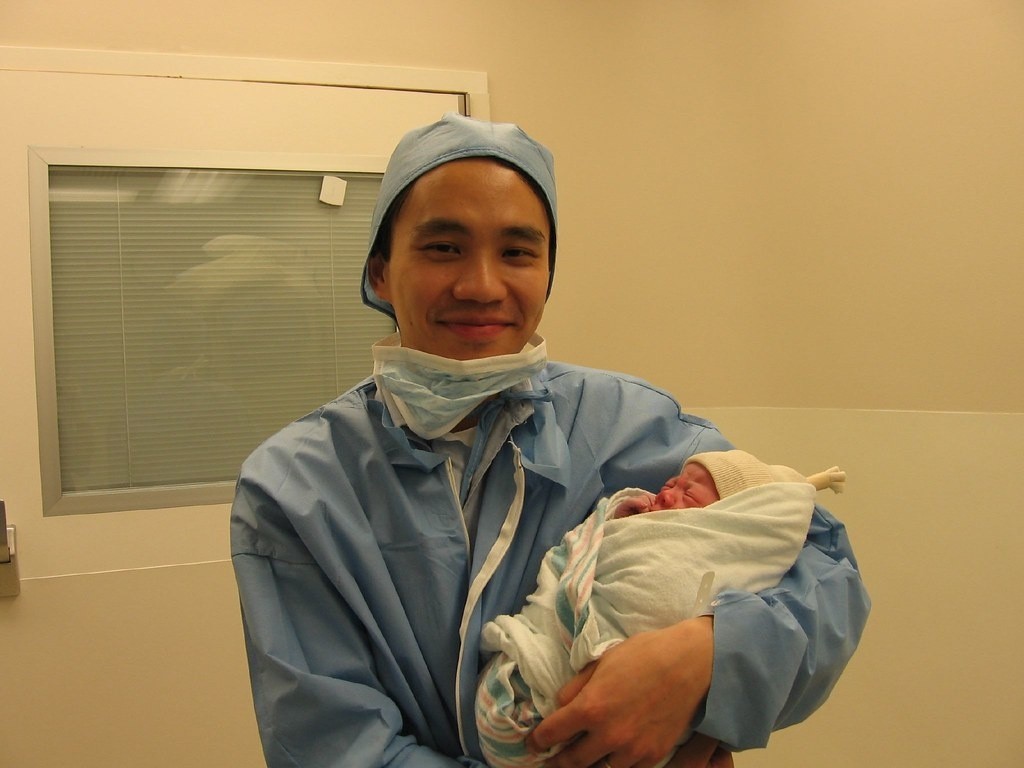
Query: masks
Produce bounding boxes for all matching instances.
[371,330,548,438]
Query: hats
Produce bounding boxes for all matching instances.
[680,450,845,497]
[354,109,563,321]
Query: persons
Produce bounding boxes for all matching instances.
[229,112,871,768]
[475,450,848,768]
